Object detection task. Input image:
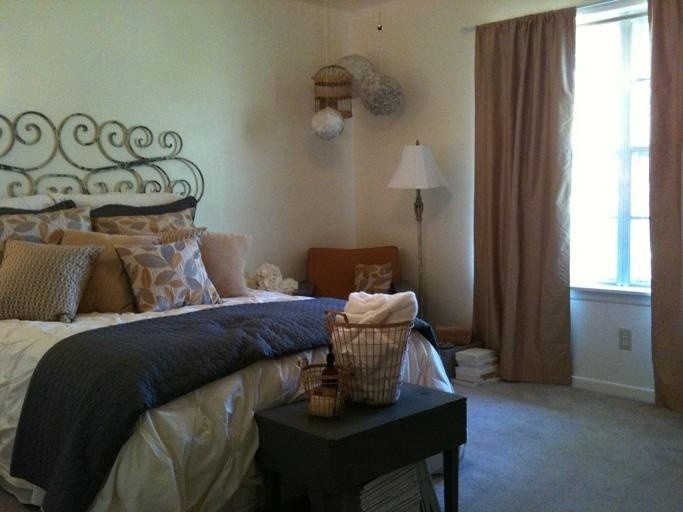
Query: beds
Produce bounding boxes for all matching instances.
[0,111,465,511]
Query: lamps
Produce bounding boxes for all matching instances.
[309,0,403,140]
[387,138,448,319]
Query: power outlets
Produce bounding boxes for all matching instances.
[618,328,632,350]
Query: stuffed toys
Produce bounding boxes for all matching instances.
[243,262,298,296]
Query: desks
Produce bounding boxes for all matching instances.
[254,376,467,511]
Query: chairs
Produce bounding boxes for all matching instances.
[293,247,400,295]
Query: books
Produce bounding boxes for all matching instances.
[360,464,423,511]
[449,348,501,389]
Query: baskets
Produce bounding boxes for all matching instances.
[298,308,415,420]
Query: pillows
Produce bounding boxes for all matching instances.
[354,261,392,295]
[0,193,251,323]
